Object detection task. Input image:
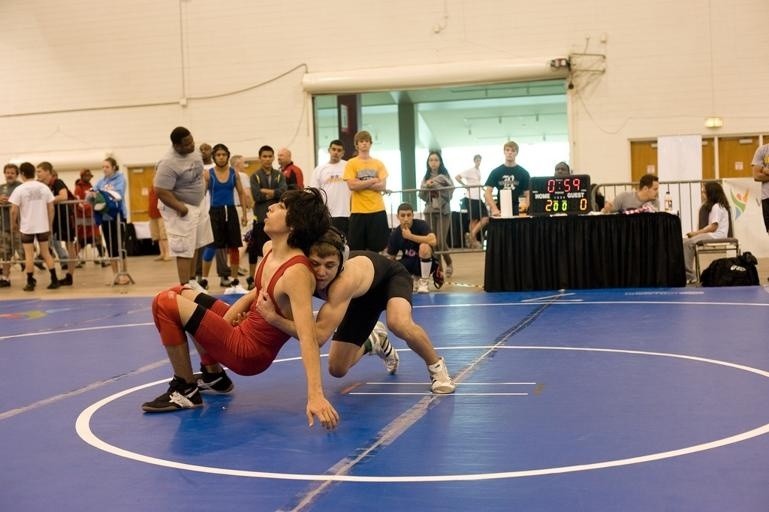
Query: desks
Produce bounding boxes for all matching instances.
[484,212,686,292]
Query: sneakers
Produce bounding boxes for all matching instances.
[687,279,697,286]
[198,276,256,290]
[58,278,72,286]
[465,233,482,249]
[141,375,204,413]
[426,356,455,394]
[196,362,234,394]
[16,258,114,270]
[0,278,10,287]
[47,280,59,289]
[368,321,399,375]
[417,278,430,293]
[23,278,36,291]
[445,265,453,278]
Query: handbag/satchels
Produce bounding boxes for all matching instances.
[698,254,760,287]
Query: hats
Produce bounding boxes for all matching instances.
[80,168,93,177]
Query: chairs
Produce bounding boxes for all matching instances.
[694,201,741,285]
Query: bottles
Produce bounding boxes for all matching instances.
[664,191,672,211]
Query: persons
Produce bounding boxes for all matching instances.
[750,142,769,234]
[311,132,391,254]
[141,185,341,430]
[483,141,531,216]
[0,157,129,293]
[148,127,307,293]
[682,180,731,283]
[388,151,455,291]
[600,174,661,212]
[553,161,570,177]
[456,155,488,249]
[232,225,455,395]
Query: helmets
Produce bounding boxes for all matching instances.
[212,144,230,168]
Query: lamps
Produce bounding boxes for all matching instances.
[706,116,723,127]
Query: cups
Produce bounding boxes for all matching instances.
[519,196,528,217]
